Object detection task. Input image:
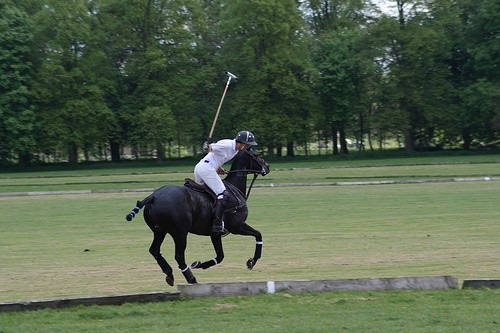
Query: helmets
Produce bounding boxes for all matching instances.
[236,130,258,146]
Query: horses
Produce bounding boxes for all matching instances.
[125,148,270,286]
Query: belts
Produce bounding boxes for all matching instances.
[204,159,209,163]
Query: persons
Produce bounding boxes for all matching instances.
[194,130,258,234]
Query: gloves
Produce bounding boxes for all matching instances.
[203,141,211,153]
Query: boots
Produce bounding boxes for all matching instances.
[210,199,228,233]
